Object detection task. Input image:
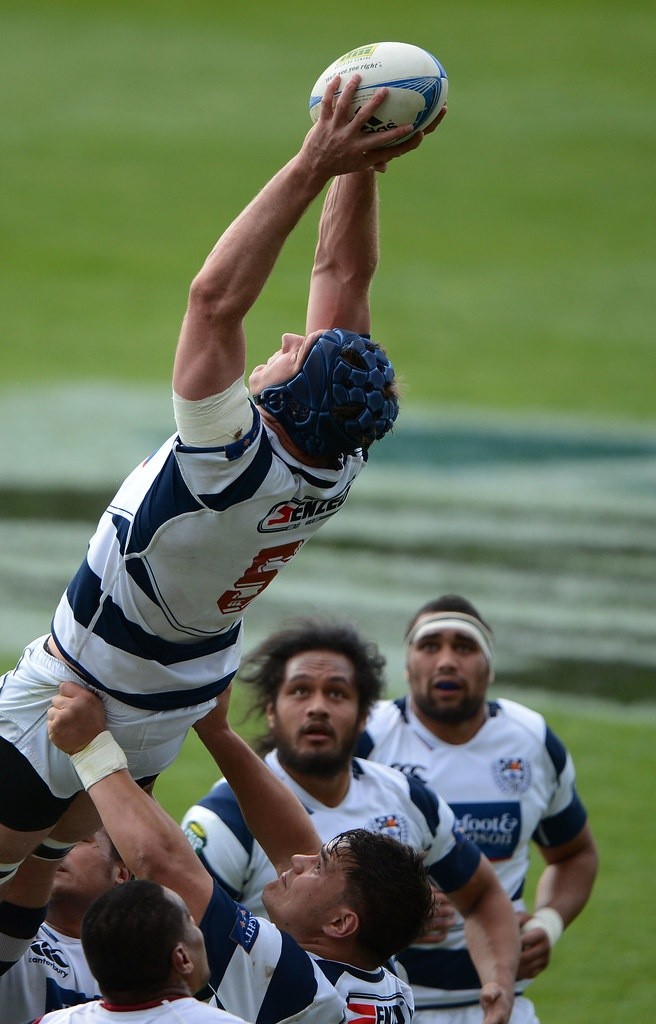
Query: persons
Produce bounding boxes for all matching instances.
[0,74,448,906]
[1,592,599,1024]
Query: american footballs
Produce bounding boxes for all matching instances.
[309,40,450,151]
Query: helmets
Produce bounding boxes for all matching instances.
[259,328,400,462]
